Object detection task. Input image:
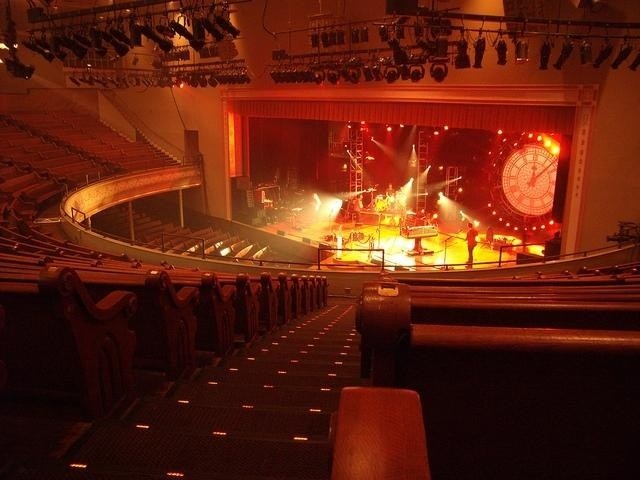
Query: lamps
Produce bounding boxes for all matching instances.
[0,0,640,88]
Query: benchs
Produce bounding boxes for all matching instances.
[0,112,639,480]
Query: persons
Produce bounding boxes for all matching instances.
[463,223,478,268]
[386,182,395,209]
[541,228,561,261]
[486,225,493,243]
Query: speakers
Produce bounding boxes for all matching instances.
[318,243,328,248]
[277,230,285,235]
[303,238,312,244]
[371,258,379,264]
[395,267,403,271]
[350,232,364,241]
